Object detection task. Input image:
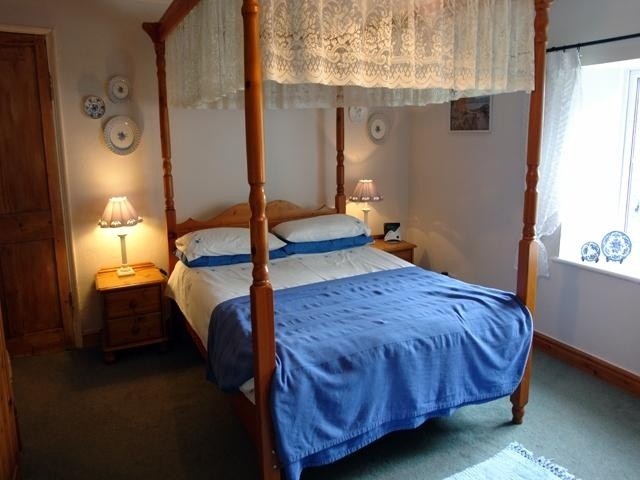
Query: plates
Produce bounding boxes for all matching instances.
[84,75,140,156]
[348,107,391,145]
[581,242,600,262]
[601,231,631,261]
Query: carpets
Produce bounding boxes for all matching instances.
[446,442,580,480]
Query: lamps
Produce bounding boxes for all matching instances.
[350,178,384,244]
[98,196,144,277]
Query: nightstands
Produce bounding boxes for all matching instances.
[95,261,169,362]
[368,238,417,266]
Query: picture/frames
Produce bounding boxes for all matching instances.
[384,223,401,244]
[448,94,493,133]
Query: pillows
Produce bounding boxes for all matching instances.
[282,236,374,255]
[175,225,288,263]
[171,247,288,269]
[272,212,372,243]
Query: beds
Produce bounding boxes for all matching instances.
[141,1,550,480]
